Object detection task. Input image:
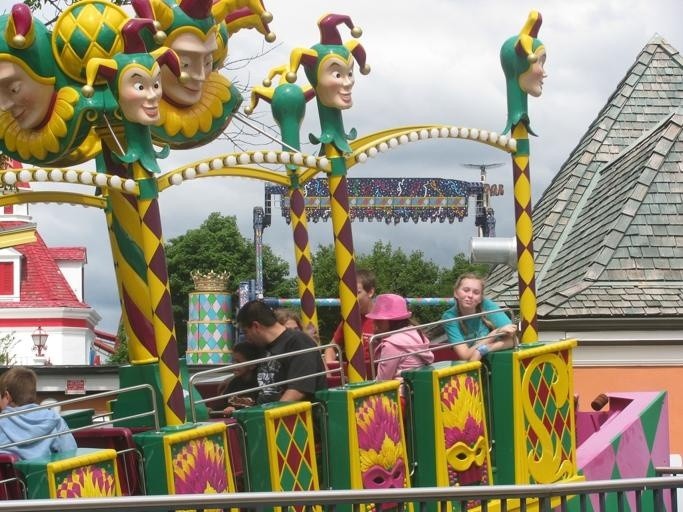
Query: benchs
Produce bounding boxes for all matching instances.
[321,359,378,378]
[427,342,492,362]
[66,426,139,496]
[190,375,350,418]
[0,452,23,503]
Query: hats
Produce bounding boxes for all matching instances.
[365,293,414,322]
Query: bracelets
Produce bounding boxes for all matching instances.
[475,344,489,357]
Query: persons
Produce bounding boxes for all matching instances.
[206,299,330,445]
[0,0,276,133]
[0,366,78,462]
[324,269,380,381]
[498,9,548,99]
[364,292,435,419]
[244,63,315,127]
[440,271,520,362]
[285,12,372,112]
